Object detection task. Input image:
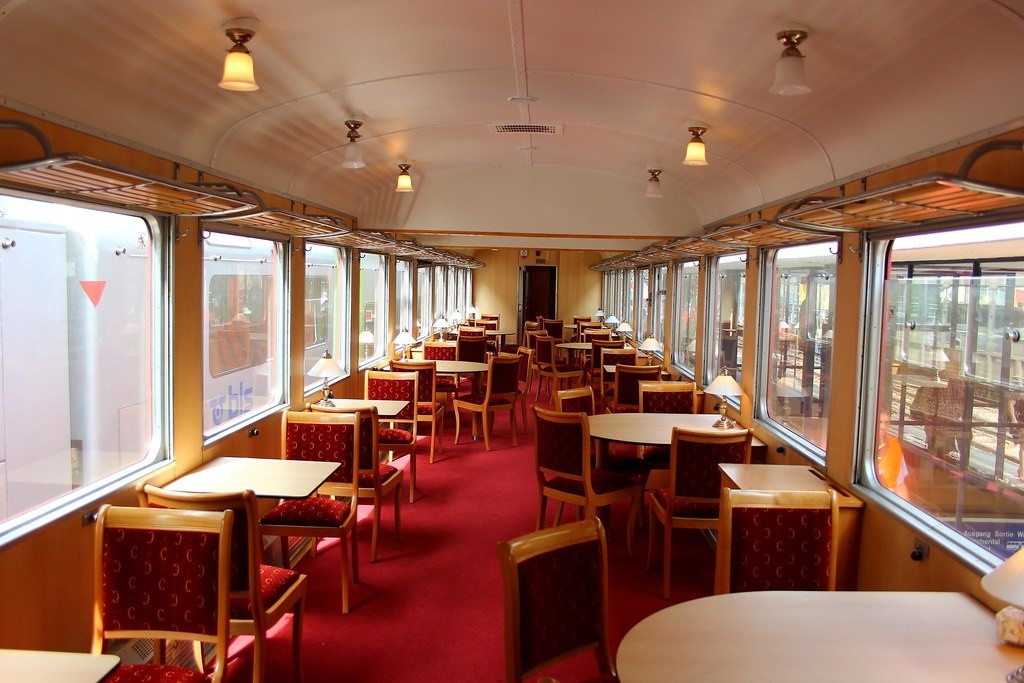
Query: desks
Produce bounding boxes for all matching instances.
[0,649,122,683]
[581,333,619,338]
[616,591,1024,683]
[588,412,768,543]
[485,329,516,352]
[555,342,635,350]
[317,399,411,416]
[565,325,608,329]
[384,359,488,439]
[161,454,342,500]
[603,365,671,376]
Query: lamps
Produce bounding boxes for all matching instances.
[393,326,418,362]
[605,314,620,335]
[450,310,464,332]
[615,319,634,347]
[307,349,347,407]
[981,546,1024,683]
[340,113,366,169]
[768,20,813,97]
[395,160,415,193]
[467,305,479,319]
[644,161,663,199]
[432,314,451,342]
[638,333,664,365]
[681,118,709,166]
[216,17,261,92]
[704,365,744,429]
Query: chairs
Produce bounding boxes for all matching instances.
[93,314,841,683]
[595,307,605,322]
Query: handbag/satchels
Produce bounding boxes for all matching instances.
[1006,395,1021,425]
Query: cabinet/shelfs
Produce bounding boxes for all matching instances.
[717,461,866,592]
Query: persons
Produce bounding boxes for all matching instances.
[1007,386,1024,482]
[920,362,973,458]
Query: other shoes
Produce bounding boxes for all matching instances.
[1017,468,1024,482]
